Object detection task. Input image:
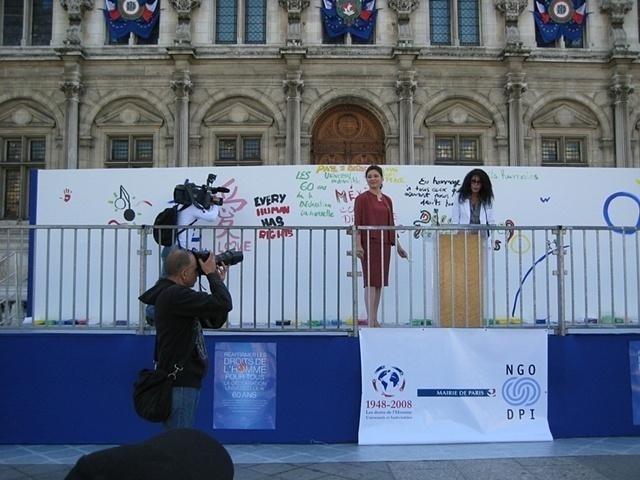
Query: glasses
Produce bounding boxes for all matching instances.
[471,180,482,185]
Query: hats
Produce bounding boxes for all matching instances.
[65,428,233,480]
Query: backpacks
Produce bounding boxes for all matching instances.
[153,204,197,249]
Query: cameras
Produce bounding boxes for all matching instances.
[193,247,244,275]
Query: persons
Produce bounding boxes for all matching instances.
[137,249,235,432]
[352,165,409,327]
[450,168,498,325]
[142,182,221,326]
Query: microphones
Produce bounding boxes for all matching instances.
[476,193,489,224]
[433,208,439,225]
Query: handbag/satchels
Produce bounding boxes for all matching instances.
[131,363,176,423]
